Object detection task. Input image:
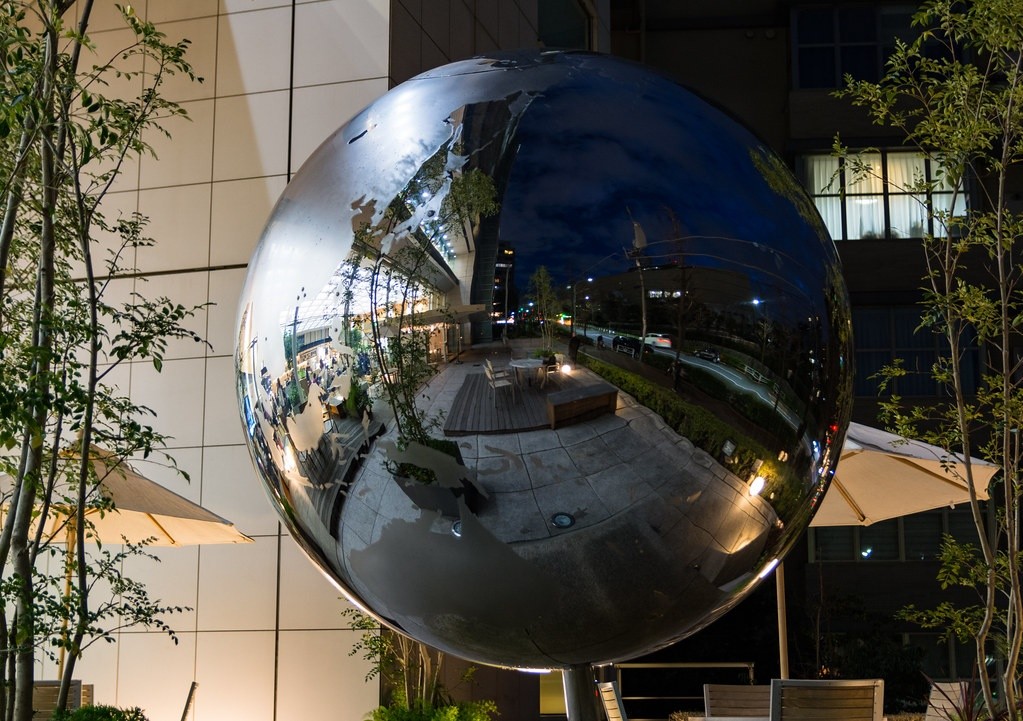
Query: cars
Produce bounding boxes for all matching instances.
[692,348,723,364]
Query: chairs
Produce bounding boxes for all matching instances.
[292,412,342,490]
[483,364,515,408]
[770,678,885,721]
[542,353,564,383]
[704,684,770,721]
[926,682,969,721]
[598,681,628,721]
[485,359,515,391]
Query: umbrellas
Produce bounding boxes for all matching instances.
[775,420,1002,681]
[0,431,255,680]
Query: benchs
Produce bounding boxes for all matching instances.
[548,381,619,429]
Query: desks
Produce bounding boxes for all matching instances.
[325,397,347,420]
[509,357,548,392]
[380,368,399,390]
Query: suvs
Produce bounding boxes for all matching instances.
[638,333,672,349]
[613,334,655,358]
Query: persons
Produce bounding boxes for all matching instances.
[252,332,367,494]
